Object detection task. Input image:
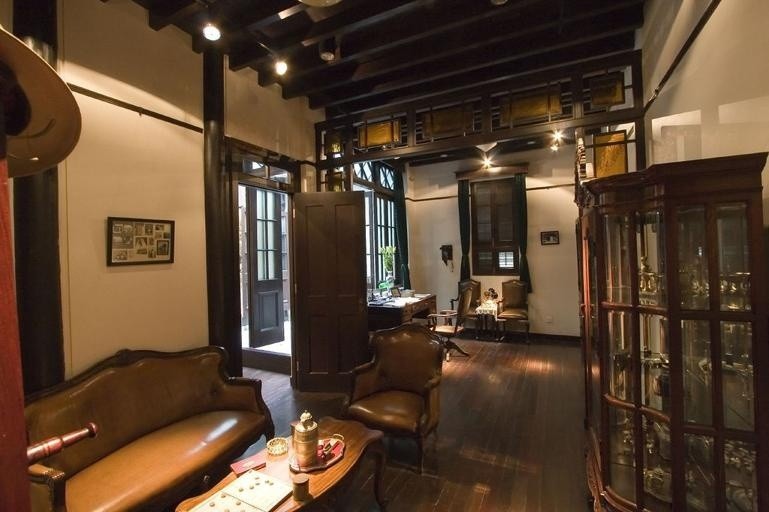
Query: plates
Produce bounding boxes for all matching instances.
[288,438,343,472]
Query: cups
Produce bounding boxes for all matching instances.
[293,474,308,500]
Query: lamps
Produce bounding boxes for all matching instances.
[191,24,221,54]
[475,141,498,153]
[550,141,560,151]
[319,39,337,61]
[379,282,389,297]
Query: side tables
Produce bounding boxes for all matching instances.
[475,300,503,342]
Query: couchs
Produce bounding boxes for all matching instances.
[342,321,445,474]
[426,286,474,362]
[24,344,275,512]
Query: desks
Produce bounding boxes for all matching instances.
[367,292,438,331]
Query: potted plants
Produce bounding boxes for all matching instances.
[380,245,396,296]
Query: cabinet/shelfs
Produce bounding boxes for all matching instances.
[573,137,769,512]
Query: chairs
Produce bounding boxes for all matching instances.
[496,279,530,344]
[450,279,481,339]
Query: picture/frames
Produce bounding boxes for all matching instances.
[106,216,175,266]
[540,231,560,245]
[390,287,402,297]
